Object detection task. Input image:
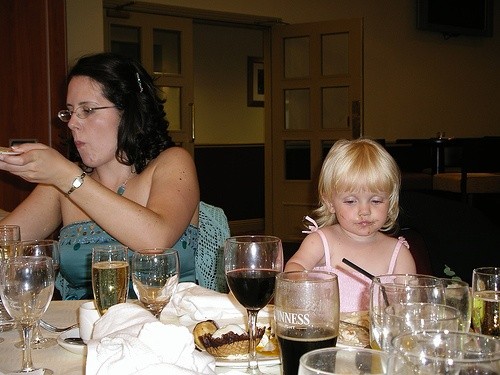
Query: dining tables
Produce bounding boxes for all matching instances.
[0,300,282,375]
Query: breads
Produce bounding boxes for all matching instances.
[193,319,219,350]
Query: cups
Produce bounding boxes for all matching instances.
[369,274,447,350]
[0,224,21,331]
[78,301,101,343]
[473,267,500,336]
[388,330,500,375]
[91,244,129,316]
[274,268,341,375]
[297,346,405,375]
[408,277,472,333]
[383,305,462,353]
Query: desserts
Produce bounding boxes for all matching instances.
[198,325,266,358]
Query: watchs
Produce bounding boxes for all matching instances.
[64,172,86,197]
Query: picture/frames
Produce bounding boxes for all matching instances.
[248,56,265,107]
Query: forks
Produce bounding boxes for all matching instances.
[40,318,80,334]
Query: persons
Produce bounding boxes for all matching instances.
[283,137,419,312]
[0,54,201,302]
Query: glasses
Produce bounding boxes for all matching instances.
[56,106,114,122]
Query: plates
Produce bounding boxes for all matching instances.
[211,318,283,366]
[57,328,87,356]
[0,147,22,156]
[332,309,369,347]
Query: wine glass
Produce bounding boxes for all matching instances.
[226,234,282,375]
[0,256,54,375]
[131,247,180,319]
[14,238,57,348]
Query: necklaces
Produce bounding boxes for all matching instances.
[118,170,135,196]
